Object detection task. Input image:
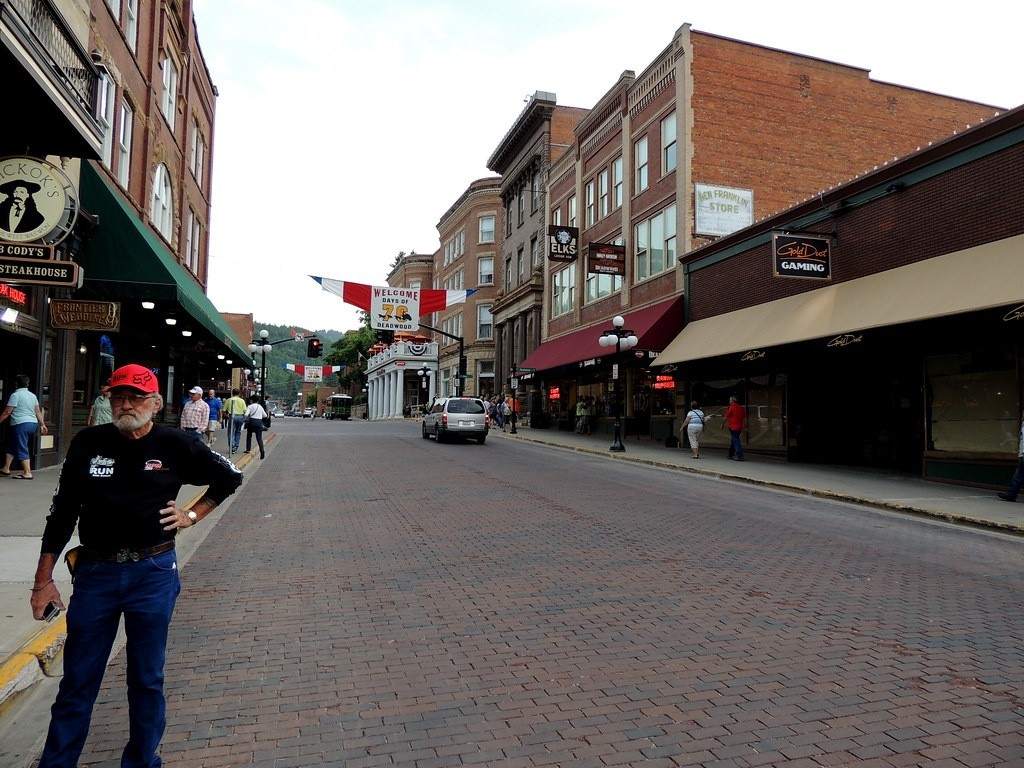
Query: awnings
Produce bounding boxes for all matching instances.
[92,159,259,369]
[648,234,1024,369]
[506,293,683,386]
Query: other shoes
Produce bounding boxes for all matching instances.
[734,456,744,462]
[260,451,264,459]
[726,455,733,459]
[243,450,250,454]
[210,438,216,445]
[206,442,210,447]
[997,492,1016,502]
[232,448,237,454]
[692,454,699,459]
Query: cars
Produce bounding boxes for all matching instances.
[269,405,313,419]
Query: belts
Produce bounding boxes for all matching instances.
[82,539,176,563]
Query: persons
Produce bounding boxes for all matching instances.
[479,392,520,432]
[222,388,246,454]
[723,396,745,463]
[679,400,705,458]
[244,394,270,459]
[596,396,601,412]
[30,364,245,768]
[179,385,210,442]
[87,372,113,426]
[576,396,594,435]
[185,387,228,447]
[0,374,48,479]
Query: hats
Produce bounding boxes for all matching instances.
[102,386,110,392]
[105,363,159,393]
[188,386,203,394]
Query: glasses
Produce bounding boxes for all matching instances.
[107,394,157,406]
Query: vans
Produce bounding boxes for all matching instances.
[421,395,491,444]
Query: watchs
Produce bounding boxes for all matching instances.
[186,509,198,526]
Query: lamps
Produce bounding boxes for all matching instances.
[827,199,846,215]
[885,181,903,193]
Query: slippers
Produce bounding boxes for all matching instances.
[11,475,33,479]
[0,469,10,475]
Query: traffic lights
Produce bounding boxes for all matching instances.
[310,338,319,358]
[318,342,324,356]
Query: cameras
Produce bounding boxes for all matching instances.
[43,601,61,623]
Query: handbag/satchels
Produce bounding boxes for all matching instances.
[244,417,250,423]
[701,421,705,432]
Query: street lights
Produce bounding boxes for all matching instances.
[248,329,272,407]
[417,362,433,413]
[598,315,638,452]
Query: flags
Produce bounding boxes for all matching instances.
[357,352,362,361]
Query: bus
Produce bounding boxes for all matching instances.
[325,392,352,420]
